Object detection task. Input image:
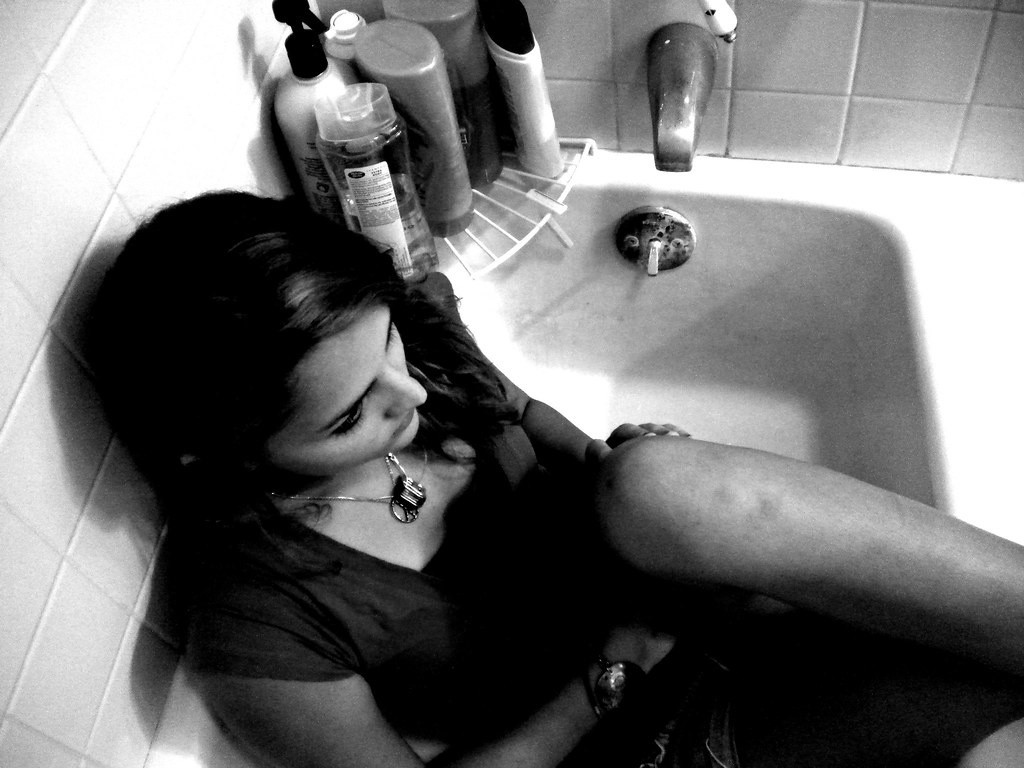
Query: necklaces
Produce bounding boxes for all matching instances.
[272,452,428,525]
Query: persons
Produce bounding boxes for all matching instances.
[86,193,1024,768]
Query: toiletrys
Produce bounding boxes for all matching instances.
[271,0,565,292]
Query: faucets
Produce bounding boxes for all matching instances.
[644,22,719,172]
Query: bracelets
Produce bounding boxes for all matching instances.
[582,652,646,716]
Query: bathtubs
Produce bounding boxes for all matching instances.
[149,151,1023,767]
